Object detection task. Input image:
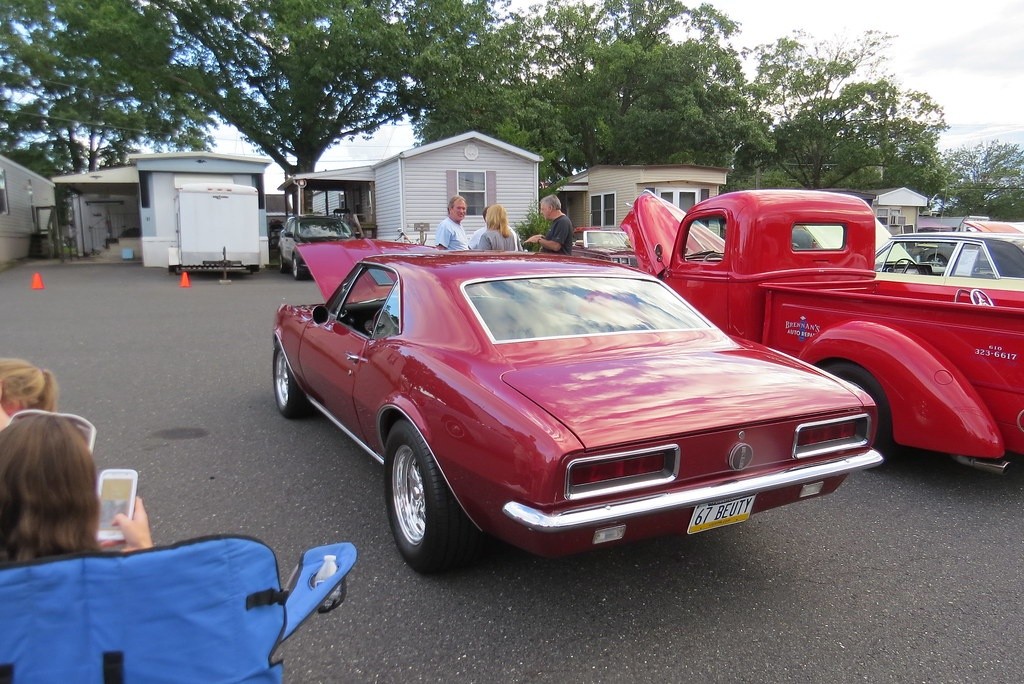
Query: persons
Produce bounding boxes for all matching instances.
[478,204,523,253]
[790,225,813,250]
[435,196,468,254]
[522,195,573,256]
[1,411,154,571]
[468,206,520,250]
[0,356,58,430]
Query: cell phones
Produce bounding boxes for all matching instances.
[95,469,138,540]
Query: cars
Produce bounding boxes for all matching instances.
[274,239,885,577]
[276,214,359,280]
[800,215,1023,292]
[570,229,639,269]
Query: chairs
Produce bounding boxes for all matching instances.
[0,535,358,684]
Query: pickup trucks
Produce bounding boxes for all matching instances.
[620,189,1023,473]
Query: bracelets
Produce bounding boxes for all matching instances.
[537,237,542,244]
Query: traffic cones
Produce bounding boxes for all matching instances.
[179,272,193,288]
[31,272,45,289]
